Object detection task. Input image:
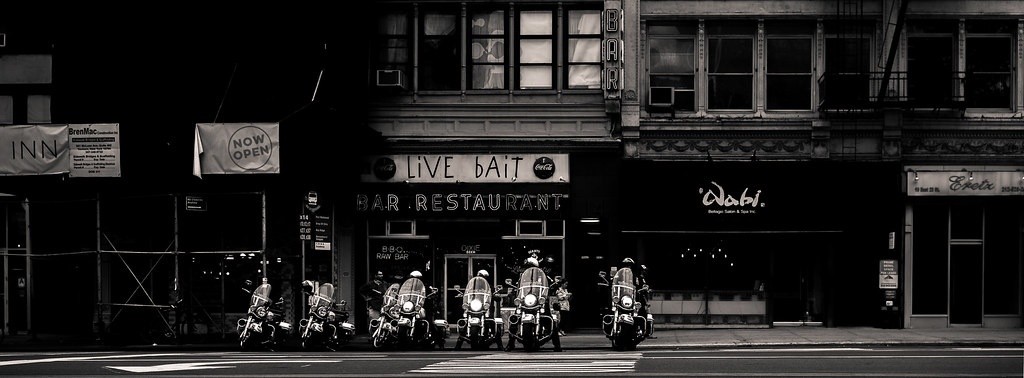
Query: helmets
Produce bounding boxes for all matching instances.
[623,257,634,264]
[477,269,489,277]
[409,271,422,278]
[524,257,540,267]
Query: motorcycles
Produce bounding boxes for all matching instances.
[368,271,450,350]
[505,267,562,352]
[237,280,294,353]
[299,280,355,352]
[598,266,658,351]
[454,276,508,351]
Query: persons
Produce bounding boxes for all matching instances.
[391,270,444,350]
[361,270,390,345]
[556,279,572,336]
[504,258,562,351]
[604,258,658,339]
[167,272,184,343]
[454,270,506,351]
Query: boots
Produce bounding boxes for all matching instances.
[504,327,518,352]
[496,340,504,351]
[551,329,562,352]
[646,319,658,339]
[454,339,463,351]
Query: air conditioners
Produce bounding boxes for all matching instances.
[648,86,674,106]
[375,69,405,90]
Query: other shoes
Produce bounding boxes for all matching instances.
[558,331,562,336]
[560,329,566,336]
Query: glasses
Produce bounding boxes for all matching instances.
[378,274,383,275]
